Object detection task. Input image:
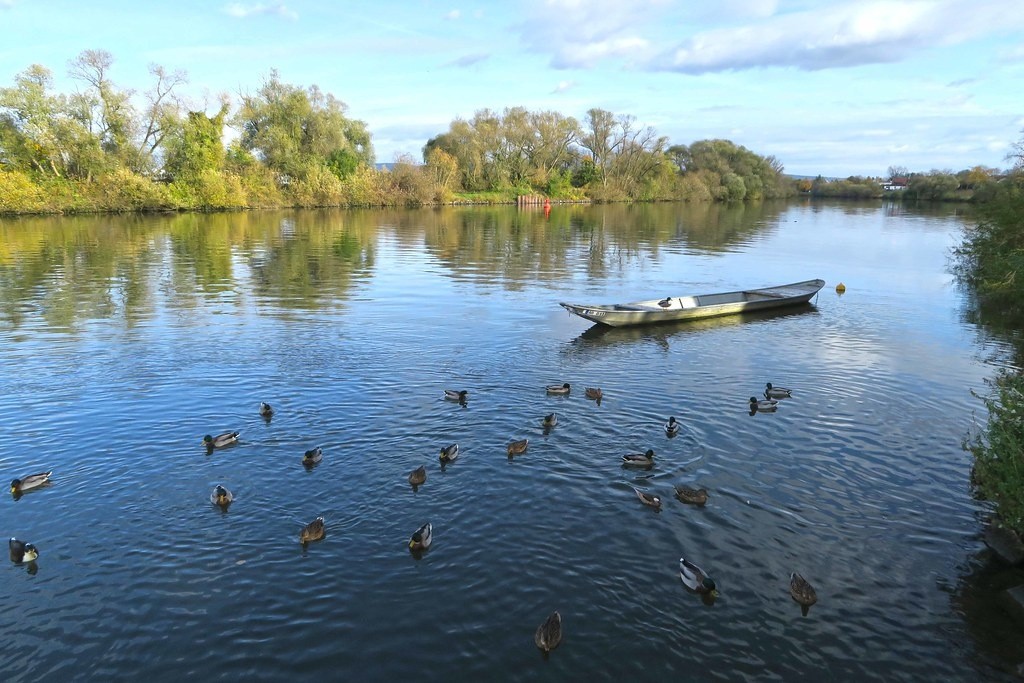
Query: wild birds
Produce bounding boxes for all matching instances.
[659,296,673,309]
[2,377,821,654]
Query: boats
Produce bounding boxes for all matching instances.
[559,279,826,328]
